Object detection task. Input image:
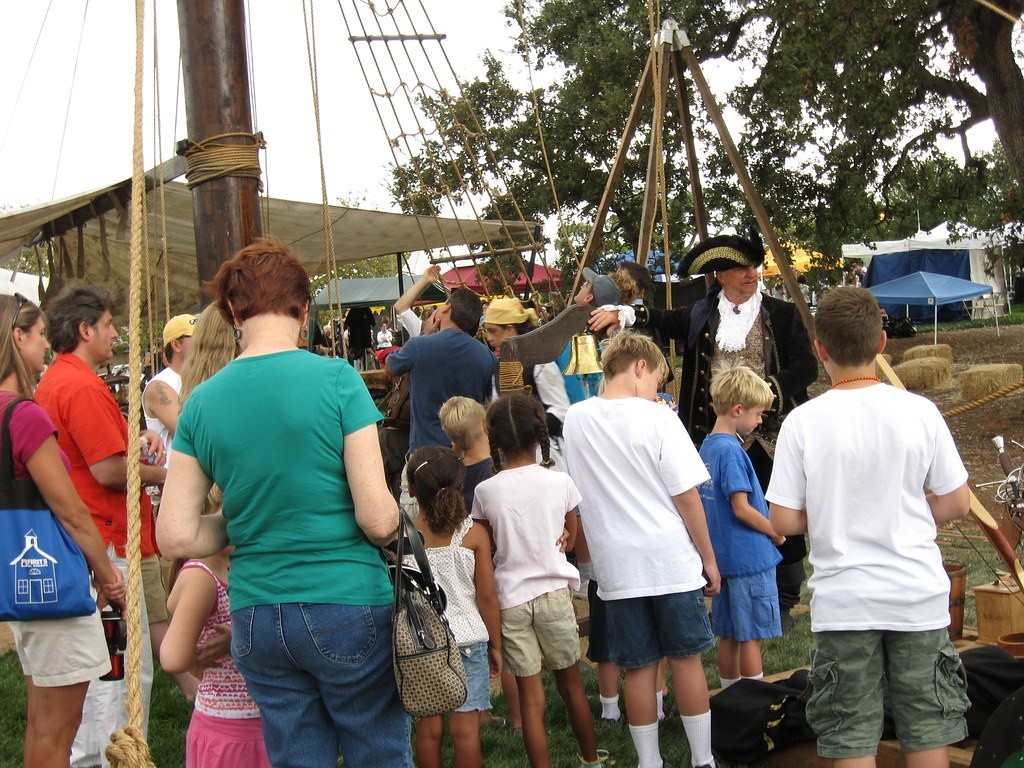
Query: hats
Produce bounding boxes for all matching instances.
[162,314,201,345]
[583,267,621,307]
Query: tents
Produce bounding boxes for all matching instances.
[757,240,845,277]
[866,270,1001,348]
[840,218,1024,324]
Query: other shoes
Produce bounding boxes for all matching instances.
[655,703,671,720]
[602,710,627,728]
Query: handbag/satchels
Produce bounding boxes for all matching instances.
[381,507,468,718]
[0,398,96,621]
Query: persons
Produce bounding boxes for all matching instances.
[693,356,786,692]
[377,236,720,768]
[763,287,973,768]
[0,292,127,768]
[588,225,820,630]
[773,275,811,310]
[142,236,416,768]
[28,289,169,768]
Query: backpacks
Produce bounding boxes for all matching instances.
[893,316,916,338]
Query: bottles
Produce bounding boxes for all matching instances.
[139,436,162,506]
[99,610,124,681]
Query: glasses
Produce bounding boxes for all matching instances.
[14,294,28,327]
[677,236,758,277]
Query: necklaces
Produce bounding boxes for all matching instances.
[832,376,880,388]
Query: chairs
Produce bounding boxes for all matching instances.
[981,294,1005,317]
[961,299,986,322]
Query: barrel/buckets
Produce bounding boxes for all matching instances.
[942,560,965,641]
[997,632,1024,663]
